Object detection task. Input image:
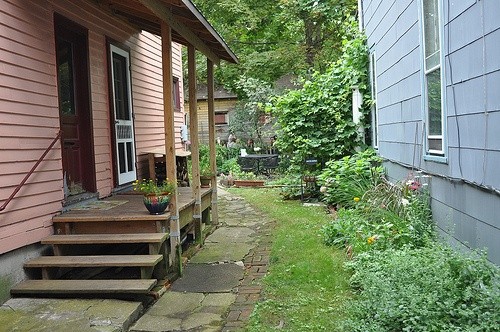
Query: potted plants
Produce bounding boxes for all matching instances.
[132,177,180,216]
